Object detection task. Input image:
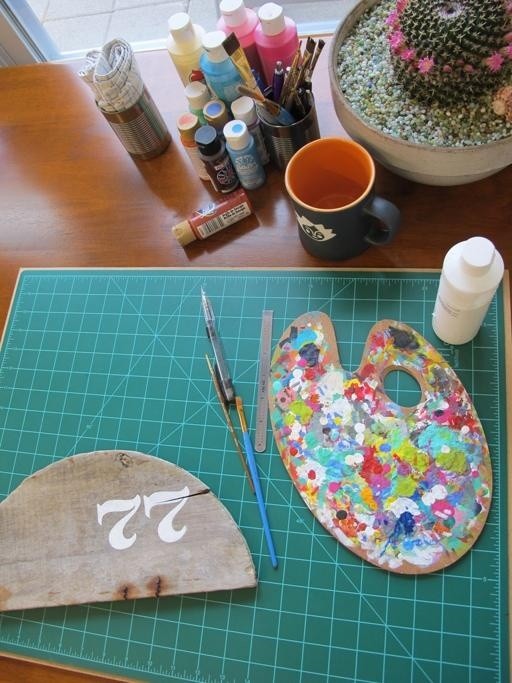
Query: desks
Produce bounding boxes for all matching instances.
[1,33,511,683]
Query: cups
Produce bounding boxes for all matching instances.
[282,135,403,263]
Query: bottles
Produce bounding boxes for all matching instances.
[214,0,266,86]
[253,1,301,89]
[230,94,273,165]
[165,10,212,88]
[170,188,255,249]
[432,236,505,346]
[198,29,250,103]
[176,80,238,194]
[223,119,269,190]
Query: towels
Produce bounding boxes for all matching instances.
[78,38,144,112]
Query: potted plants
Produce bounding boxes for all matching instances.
[328,0,512,186]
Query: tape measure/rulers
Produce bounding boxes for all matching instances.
[254,310,274,454]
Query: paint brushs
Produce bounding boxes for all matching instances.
[203,353,279,568]
[222,32,326,126]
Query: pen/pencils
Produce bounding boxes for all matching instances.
[199,284,235,402]
